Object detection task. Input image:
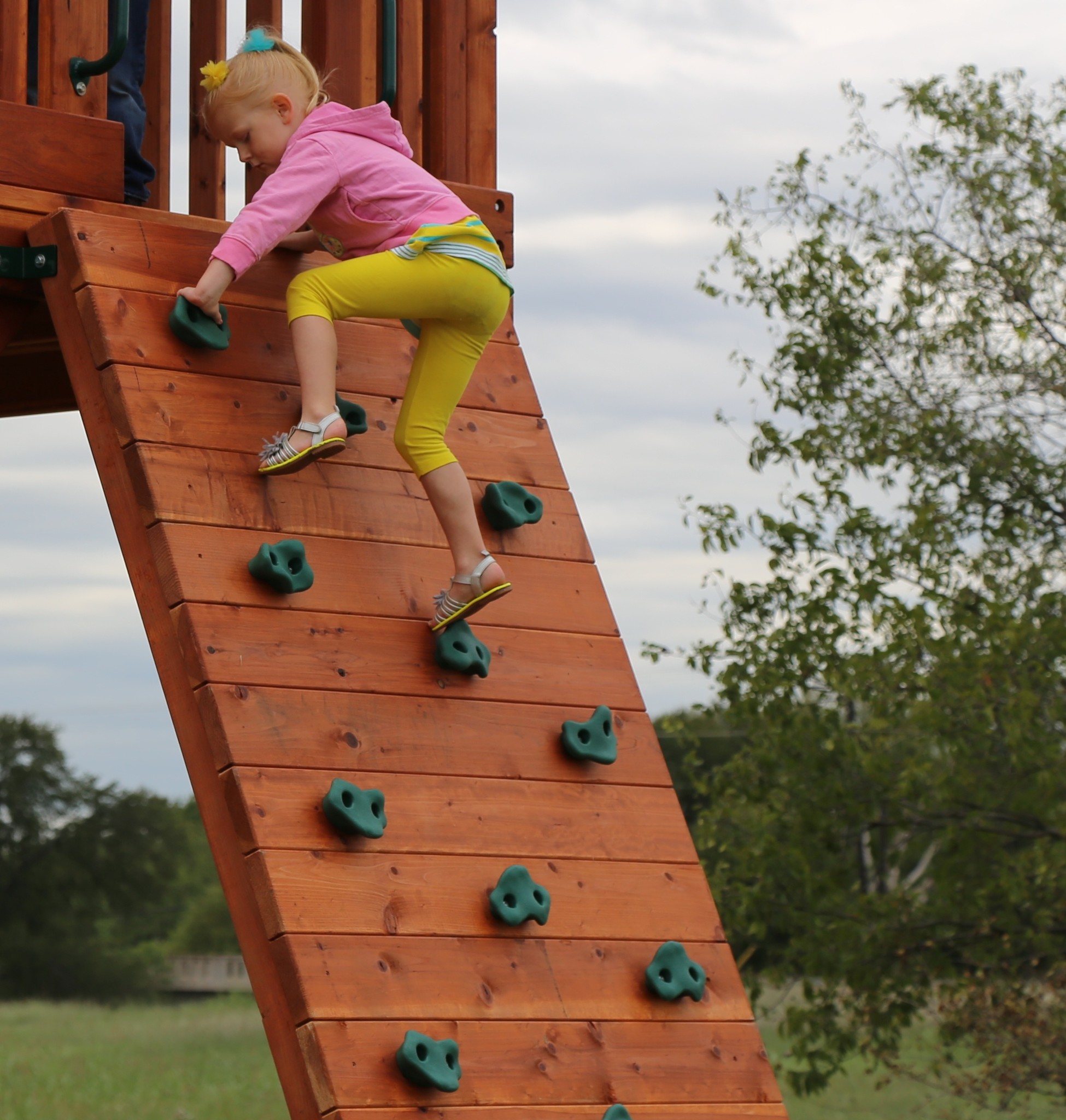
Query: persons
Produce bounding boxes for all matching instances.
[177,24,515,632]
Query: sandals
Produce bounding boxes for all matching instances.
[257,411,347,476]
[427,548,513,633]
